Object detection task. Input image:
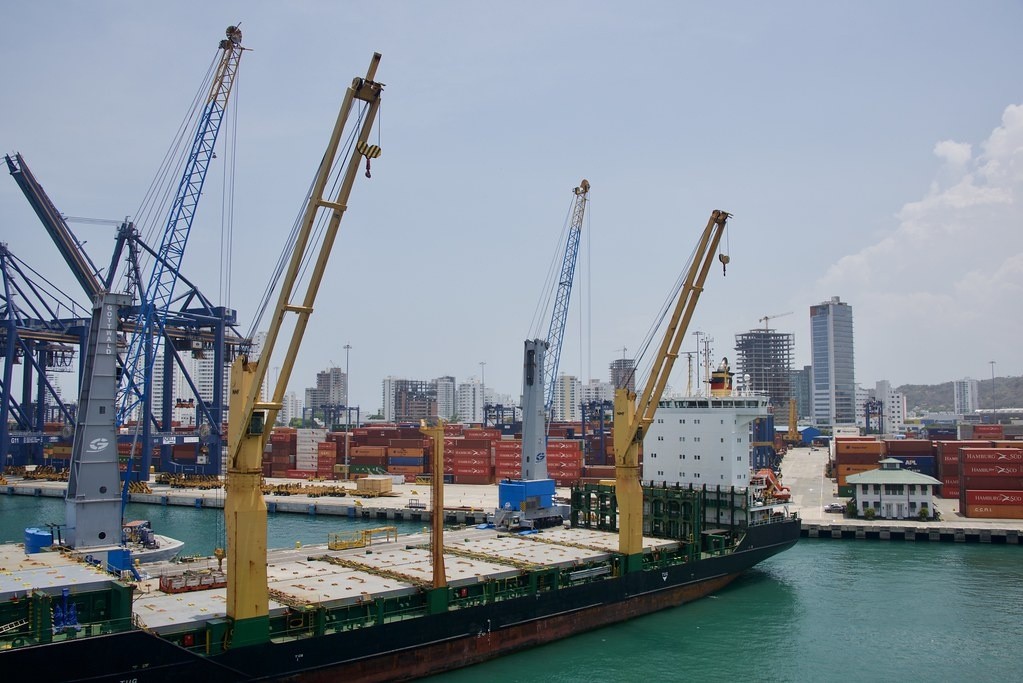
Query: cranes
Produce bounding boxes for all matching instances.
[759,310,794,333]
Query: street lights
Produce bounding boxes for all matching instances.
[342,344,353,481]
[692,331,704,389]
[987,359,999,424]
[478,361,488,430]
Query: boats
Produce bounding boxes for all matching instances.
[0,21,802,683]
[45,521,184,563]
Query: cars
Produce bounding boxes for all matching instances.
[823,503,846,513]
